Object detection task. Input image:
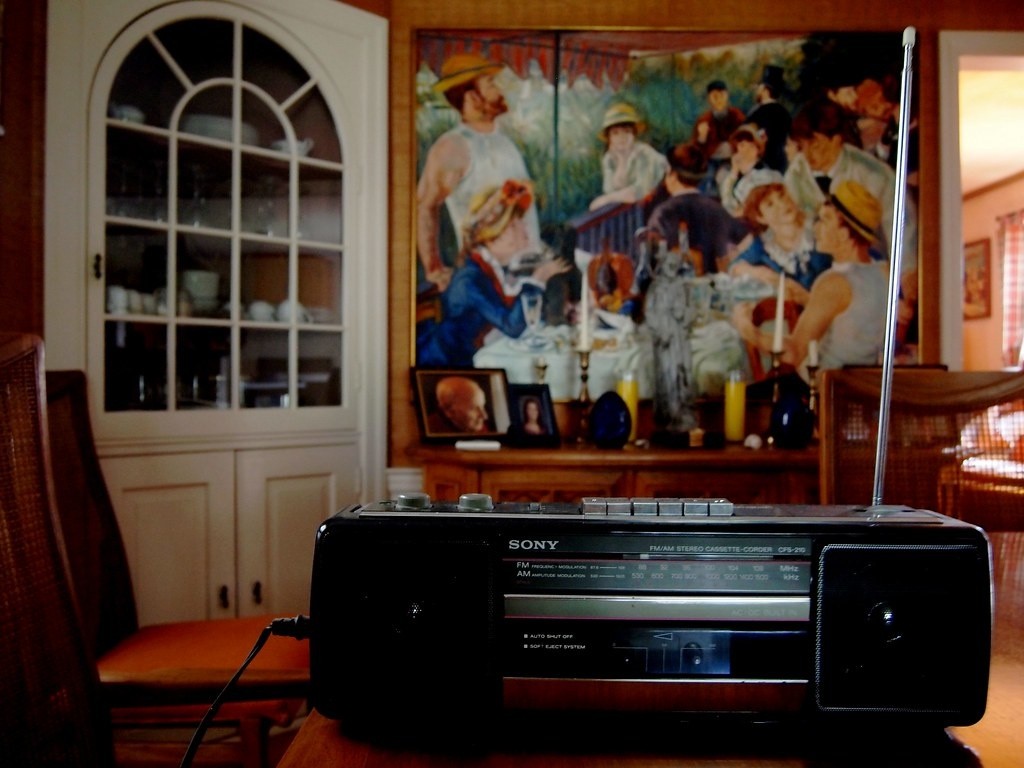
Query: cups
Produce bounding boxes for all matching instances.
[266,137,315,157]
[105,269,340,325]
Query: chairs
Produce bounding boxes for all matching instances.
[43,365,310,767]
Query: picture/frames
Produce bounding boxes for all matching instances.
[410,363,513,445]
[389,1,945,463]
[505,382,561,449]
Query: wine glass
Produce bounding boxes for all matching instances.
[102,159,293,236]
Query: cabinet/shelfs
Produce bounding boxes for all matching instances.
[0,1,391,626]
[407,444,820,501]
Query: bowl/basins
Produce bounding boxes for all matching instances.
[182,113,261,146]
[116,105,144,122]
